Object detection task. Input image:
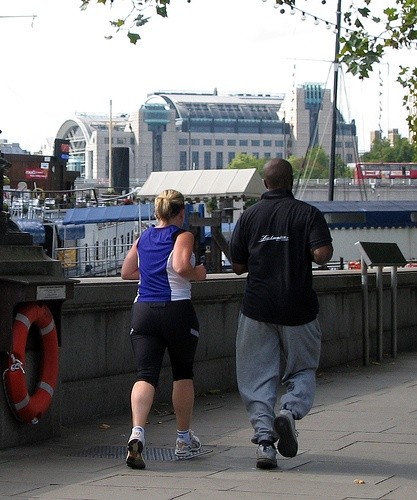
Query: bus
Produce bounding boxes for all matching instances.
[354,162,417,184]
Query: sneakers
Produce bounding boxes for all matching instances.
[125,431,145,469]
[256,445,277,466]
[175,430,200,456]
[274,410,299,457]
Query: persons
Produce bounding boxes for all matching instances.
[229,159,334,469]
[121,189,207,469]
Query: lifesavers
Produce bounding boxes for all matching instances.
[32,187,46,201]
[6,306,60,423]
